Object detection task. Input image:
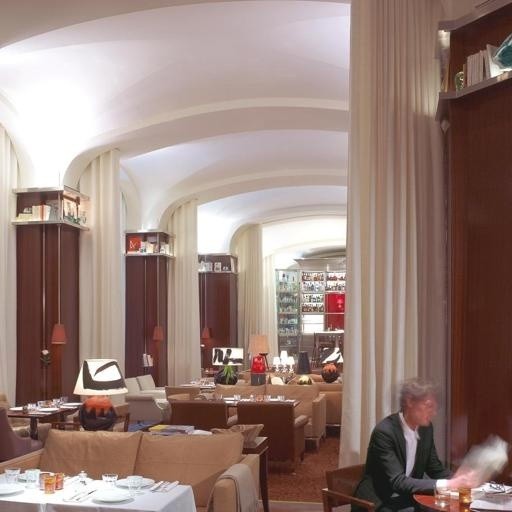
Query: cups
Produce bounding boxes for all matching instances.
[212,392,287,403]
[6,396,85,416]
[0,465,181,504]
[434,485,473,512]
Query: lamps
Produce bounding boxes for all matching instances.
[273,350,295,373]
[319,346,344,383]
[212,346,244,387]
[73,359,128,431]
[247,335,270,386]
[152,255,163,342]
[201,273,211,339]
[50,223,67,345]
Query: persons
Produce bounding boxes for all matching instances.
[349,376,487,511]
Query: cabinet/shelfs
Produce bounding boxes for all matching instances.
[275,268,346,357]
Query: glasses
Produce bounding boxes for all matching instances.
[489,480,505,493]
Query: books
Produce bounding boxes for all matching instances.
[148,423,194,433]
[150,430,194,436]
[461,42,503,88]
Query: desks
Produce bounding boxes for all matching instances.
[412,482,511,512]
[0,467,191,512]
[6,399,83,440]
[76,431,269,511]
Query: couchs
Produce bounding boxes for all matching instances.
[166,384,328,447]
[124,375,166,421]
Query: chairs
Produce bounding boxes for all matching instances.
[170,400,237,429]
[236,401,308,466]
[1,428,260,512]
[1,396,42,460]
[321,464,376,511]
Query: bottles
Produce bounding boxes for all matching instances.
[300,270,345,313]
